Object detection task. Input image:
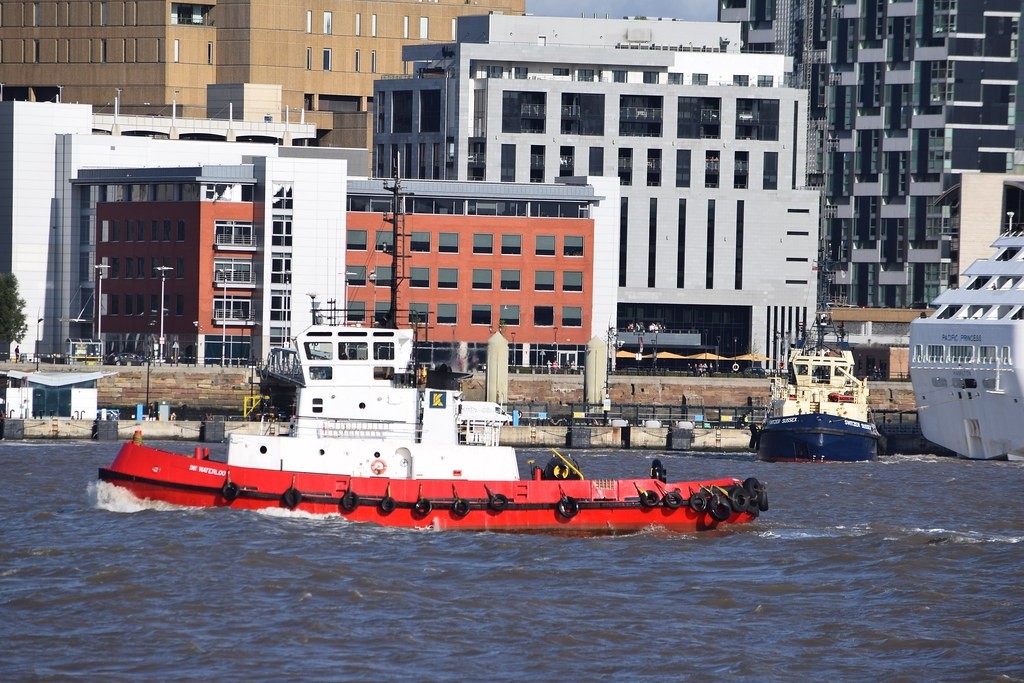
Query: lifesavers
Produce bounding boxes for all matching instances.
[370,459,388,475]
[639,490,659,508]
[730,477,769,512]
[380,497,396,512]
[221,482,240,501]
[705,494,731,522]
[450,498,470,516]
[282,487,302,508]
[341,491,360,512]
[557,496,580,518]
[414,498,432,516]
[664,492,683,509]
[489,493,507,511]
[688,492,707,512]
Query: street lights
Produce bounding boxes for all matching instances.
[650,339,656,372]
[552,327,558,366]
[732,336,738,365]
[216,269,237,367]
[716,336,722,374]
[309,294,317,325]
[93,264,112,341]
[614,334,619,355]
[510,332,516,367]
[153,266,174,363]
[35,318,43,374]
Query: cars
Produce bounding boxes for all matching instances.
[106,352,145,366]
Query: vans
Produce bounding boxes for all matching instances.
[744,367,766,379]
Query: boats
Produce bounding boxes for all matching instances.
[94,152,774,533]
[755,250,889,462]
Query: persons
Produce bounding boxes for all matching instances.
[148,403,155,420]
[553,361,559,373]
[571,361,576,374]
[15,345,20,362]
[688,362,714,377]
[628,322,645,333]
[650,459,667,483]
[649,323,664,333]
